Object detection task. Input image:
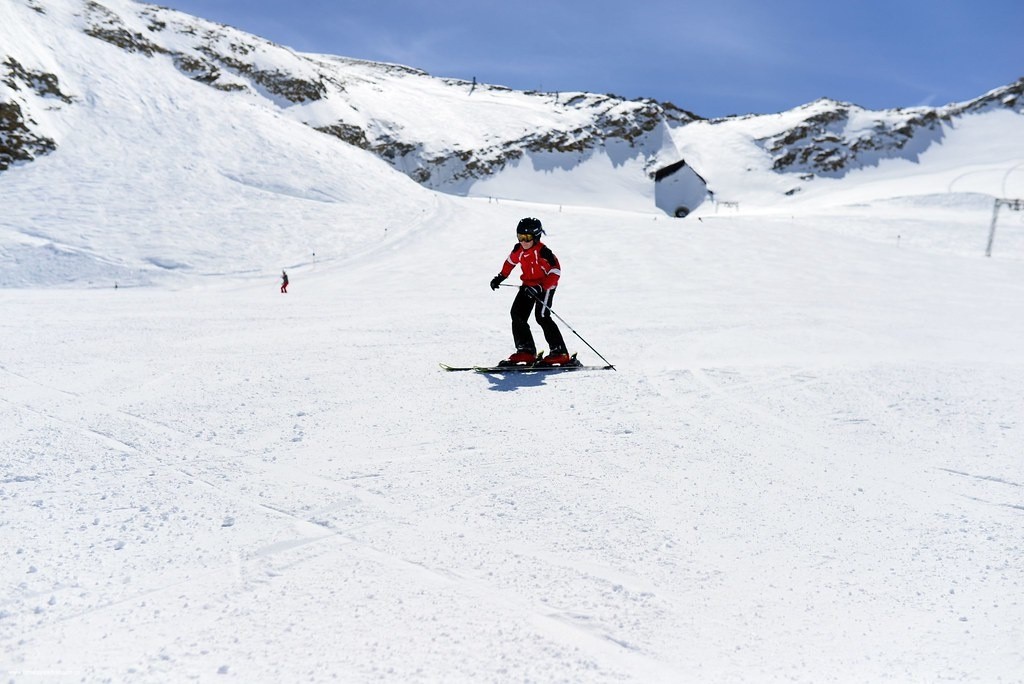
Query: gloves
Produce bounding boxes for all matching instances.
[490,275,506,291]
[522,284,544,300]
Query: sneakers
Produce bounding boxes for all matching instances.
[543,345,568,364]
[510,350,536,364]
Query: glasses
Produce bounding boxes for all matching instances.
[517,235,533,241]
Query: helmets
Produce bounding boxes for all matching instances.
[516,219,541,248]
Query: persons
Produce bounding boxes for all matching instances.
[280,272,289,293]
[490,217,570,366]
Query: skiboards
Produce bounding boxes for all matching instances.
[439,362,620,373]
